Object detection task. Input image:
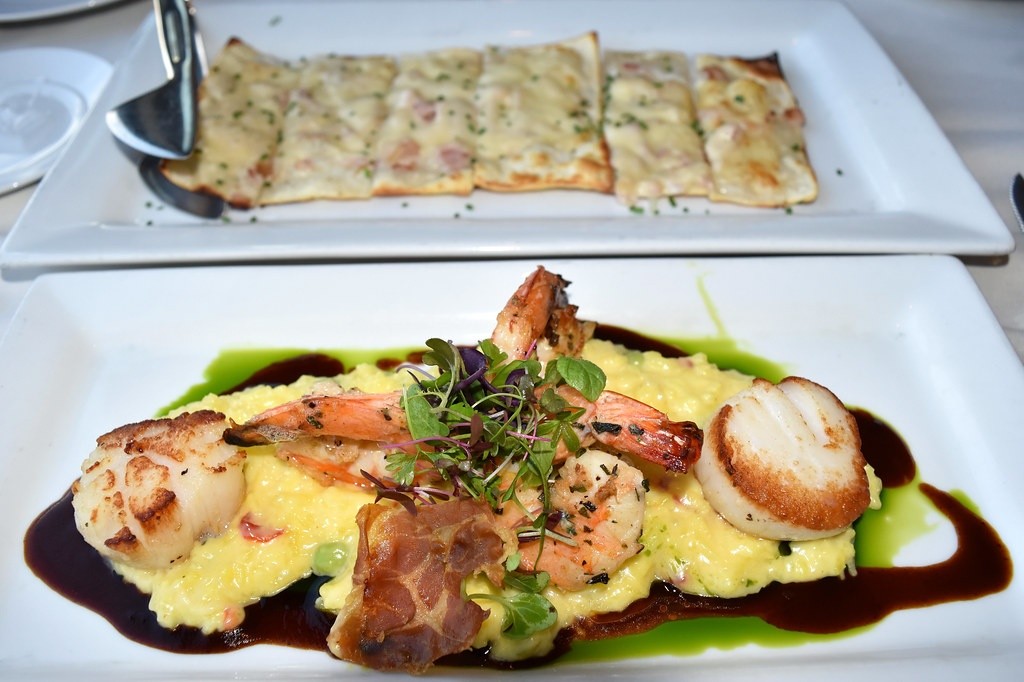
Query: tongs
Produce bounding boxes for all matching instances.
[104,1,224,220]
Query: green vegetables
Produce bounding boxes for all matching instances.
[383,337,608,638]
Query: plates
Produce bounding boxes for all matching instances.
[0,1,116,21]
[0,46,113,196]
[0,253,1024,682]
[0,1,1016,268]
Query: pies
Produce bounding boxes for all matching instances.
[156,33,821,208]
